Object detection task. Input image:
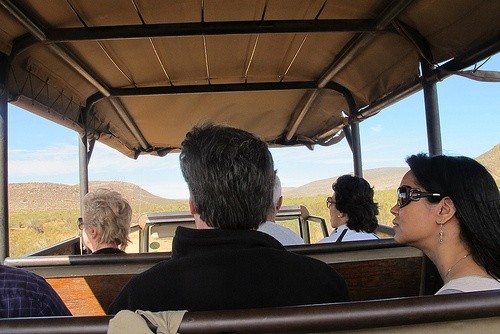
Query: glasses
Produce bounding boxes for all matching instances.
[397,185,441,208]
[327,197,336,208]
[77,218,84,230]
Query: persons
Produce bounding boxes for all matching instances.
[317,175,381,243]
[390,153,500,296]
[106,124,351,315]
[77,188,132,255]
[0,264,73,318]
[250,175,306,246]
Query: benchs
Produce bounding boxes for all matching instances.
[1,288,500,334]
[2,237,445,316]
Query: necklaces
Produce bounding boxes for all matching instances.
[442,250,472,281]
